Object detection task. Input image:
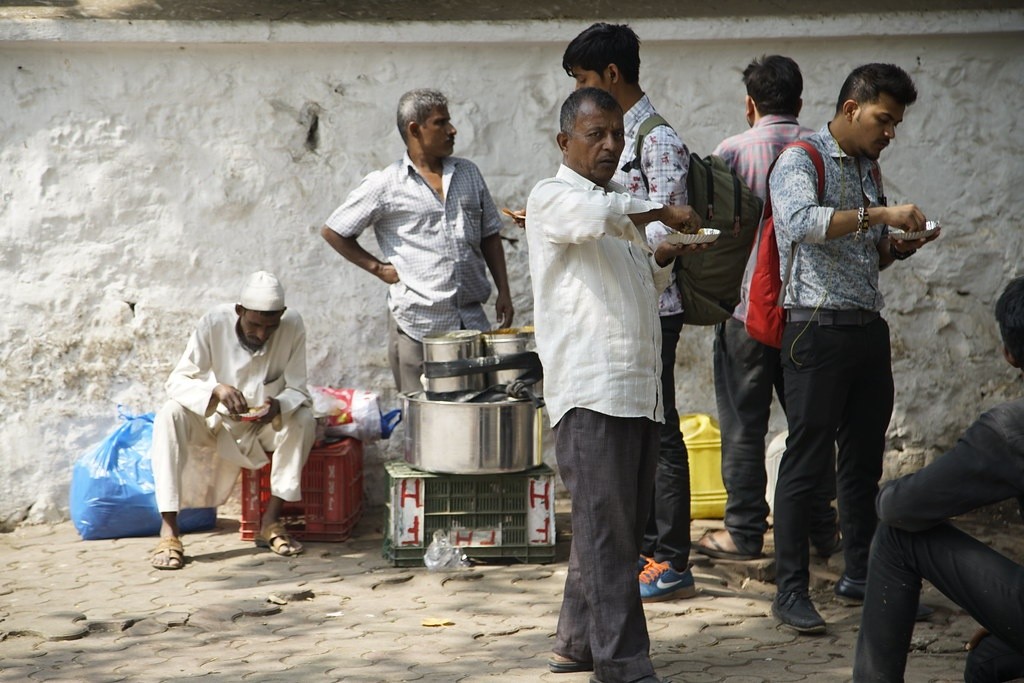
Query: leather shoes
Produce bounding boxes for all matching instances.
[834,574,934,619]
[772,589,826,631]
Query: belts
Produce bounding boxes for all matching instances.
[785,309,880,326]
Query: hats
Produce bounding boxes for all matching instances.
[237,270,286,310]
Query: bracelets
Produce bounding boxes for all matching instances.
[855,207,870,241]
[889,244,916,260]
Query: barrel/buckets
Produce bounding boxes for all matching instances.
[679,412,728,519]
[422,326,539,402]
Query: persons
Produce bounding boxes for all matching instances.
[152,271,316,570]
[769,64,940,635]
[321,87,514,407]
[691,55,844,560]
[525,88,718,683]
[851,277,1024,683]
[514,22,696,602]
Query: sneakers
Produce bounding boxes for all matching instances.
[639,559,697,602]
[637,555,654,571]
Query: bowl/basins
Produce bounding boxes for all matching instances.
[396,390,543,475]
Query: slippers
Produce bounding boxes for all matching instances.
[255,524,302,555]
[809,531,841,564]
[549,656,594,673]
[153,537,184,569]
[691,533,756,560]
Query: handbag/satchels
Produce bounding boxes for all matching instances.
[70,400,217,540]
[307,384,404,446]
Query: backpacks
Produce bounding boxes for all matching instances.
[741,143,881,348]
[622,114,763,325]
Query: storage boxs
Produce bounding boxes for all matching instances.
[381,460,557,567]
[239,439,365,545]
